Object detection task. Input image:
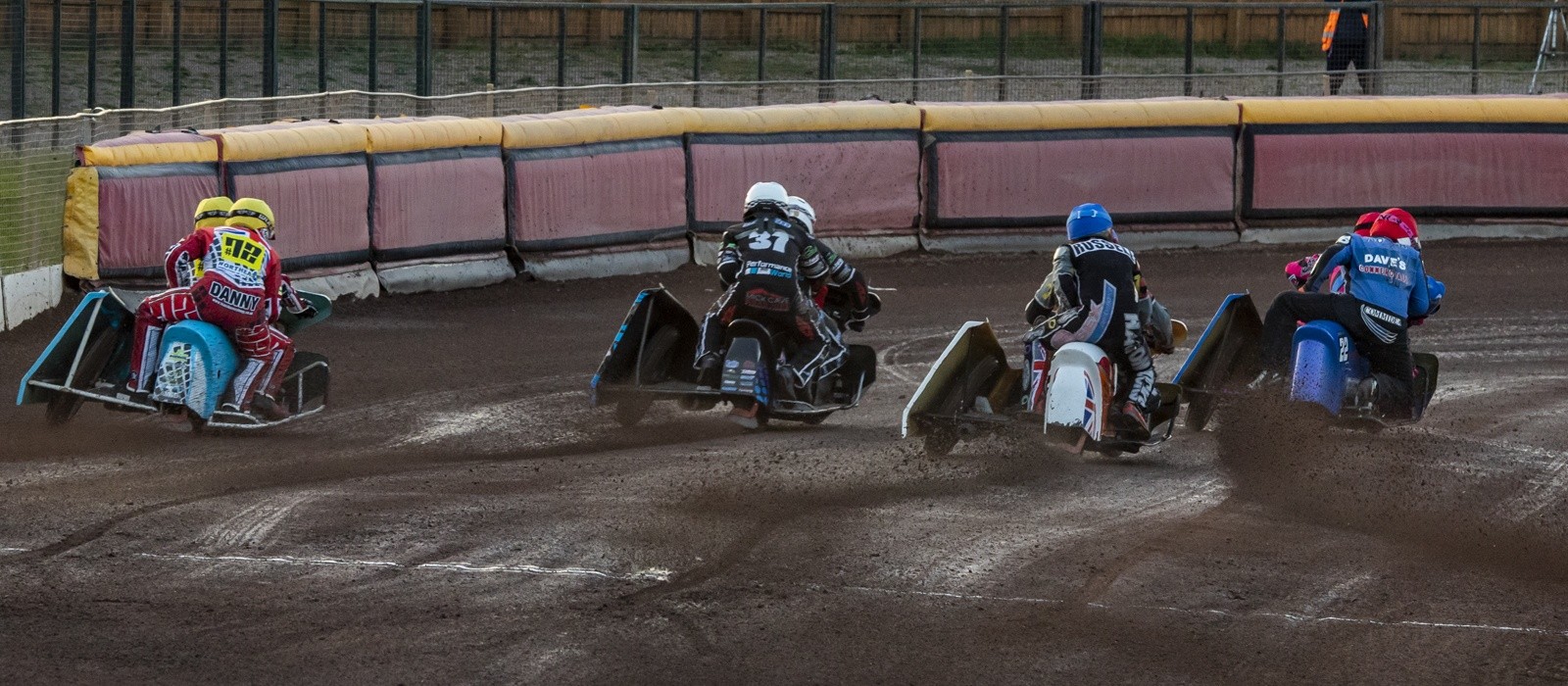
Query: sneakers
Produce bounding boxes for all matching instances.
[1354,378,1379,417]
[1246,369,1285,391]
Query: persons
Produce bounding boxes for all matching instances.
[1260,205,1447,414]
[113,189,294,425]
[1020,200,1174,440]
[694,181,872,404]
[1321,0,1369,95]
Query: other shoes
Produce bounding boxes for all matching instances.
[776,365,797,408]
[1121,401,1152,442]
[126,379,150,393]
[251,394,289,421]
[1010,402,1030,420]
[792,362,813,405]
[218,407,266,424]
[696,360,719,387]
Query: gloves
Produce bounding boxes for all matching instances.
[292,305,319,320]
[1164,347,1174,355]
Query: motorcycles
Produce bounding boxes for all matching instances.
[586,273,880,427]
[1174,264,1445,451]
[904,300,1184,458]
[14,286,321,422]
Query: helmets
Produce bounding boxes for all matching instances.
[1353,212,1380,237]
[1111,229,1119,244]
[787,196,817,235]
[1370,207,1422,252]
[193,196,234,228]
[1066,203,1119,244]
[743,182,789,218]
[225,197,276,242]
